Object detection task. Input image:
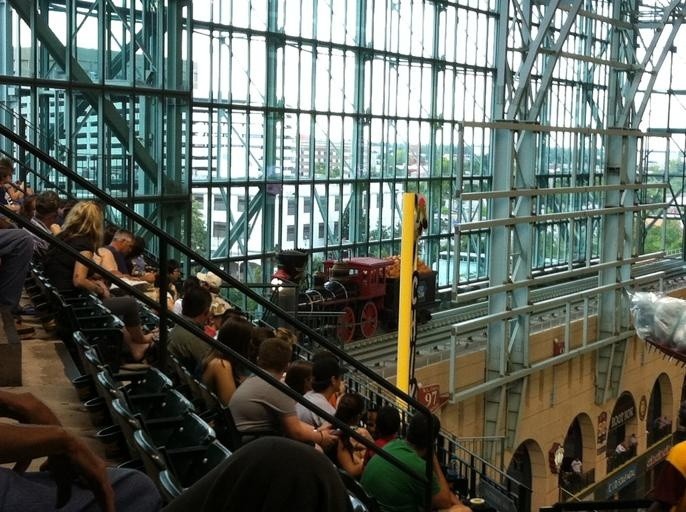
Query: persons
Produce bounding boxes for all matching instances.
[631,433,637,456]
[0,387,162,512]
[163,437,354,512]
[154,259,340,453]
[615,441,627,464]
[289,350,471,512]
[571,457,583,486]
[559,469,570,500]
[0,159,154,362]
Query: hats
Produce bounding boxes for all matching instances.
[197,271,222,289]
[311,358,349,377]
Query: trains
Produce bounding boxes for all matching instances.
[266,247,438,345]
